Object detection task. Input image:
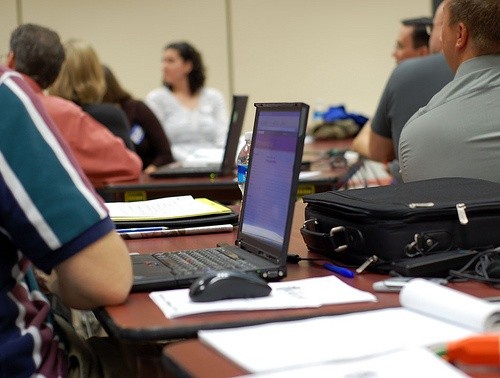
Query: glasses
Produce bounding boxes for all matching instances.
[425,22,441,36]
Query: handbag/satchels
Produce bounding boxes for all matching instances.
[300,177,500,276]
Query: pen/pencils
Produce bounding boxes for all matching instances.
[314,259,352,278]
[117,226,168,234]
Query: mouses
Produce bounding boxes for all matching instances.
[188,268,273,303]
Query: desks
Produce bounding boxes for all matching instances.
[95,173,246,207]
[158,298,500,378]
[93,200,500,378]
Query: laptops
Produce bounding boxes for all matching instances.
[130,102,310,294]
[148,96,249,180]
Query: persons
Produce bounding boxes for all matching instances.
[144,41,230,160]
[397,1,499,185]
[46,39,136,153]
[0,63,133,378]
[6,23,143,189]
[349,17,433,160]
[368,1,455,179]
[101,64,176,176]
